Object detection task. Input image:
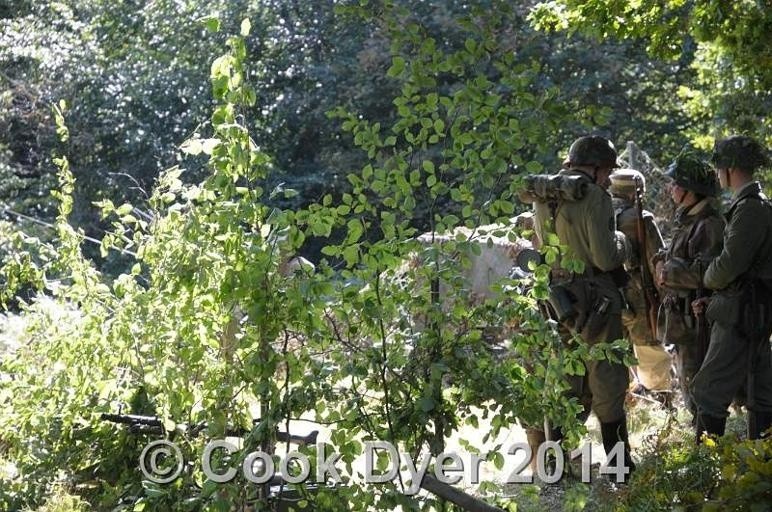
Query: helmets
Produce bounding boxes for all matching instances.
[609,169,645,194]
[711,135,763,168]
[662,159,716,196]
[562,135,621,168]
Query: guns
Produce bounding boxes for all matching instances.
[632,176,663,344]
[691,258,708,447]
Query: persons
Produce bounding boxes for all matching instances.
[650,158,725,426]
[606,167,674,413]
[687,135,771,446]
[503,211,546,475]
[533,134,634,479]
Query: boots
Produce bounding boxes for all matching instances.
[747,410,771,440]
[601,415,636,482]
[543,402,573,479]
[695,409,726,446]
